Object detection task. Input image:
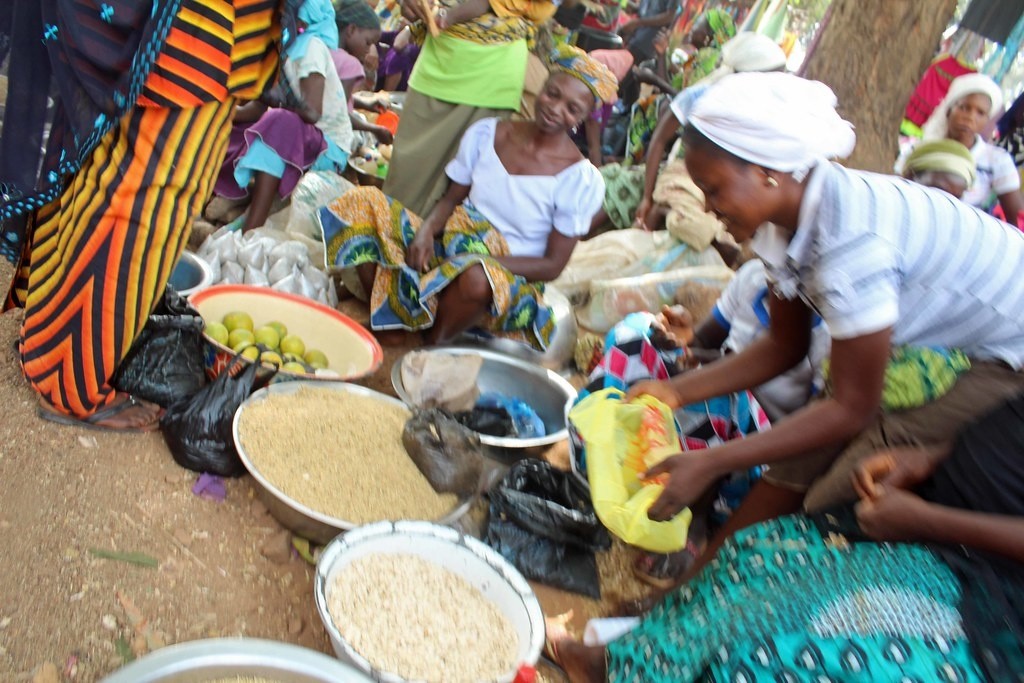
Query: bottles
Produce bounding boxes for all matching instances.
[354,154,389,178]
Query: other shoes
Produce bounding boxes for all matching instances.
[540,617,606,683]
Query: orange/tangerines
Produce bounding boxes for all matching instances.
[204,311,329,375]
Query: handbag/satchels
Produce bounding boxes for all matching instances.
[596,522,676,618]
[570,387,692,553]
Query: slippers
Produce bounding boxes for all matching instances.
[36,391,166,434]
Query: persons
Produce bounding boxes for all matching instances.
[0,0,1024,682]
[316,40,634,351]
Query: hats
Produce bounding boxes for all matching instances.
[687,71,856,180]
[903,140,978,193]
[547,43,619,124]
[718,30,787,74]
[704,8,737,41]
[945,72,1005,120]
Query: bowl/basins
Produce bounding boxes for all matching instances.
[170,250,213,297]
[187,284,384,392]
[231,379,475,545]
[95,636,376,683]
[390,344,578,448]
[313,519,546,683]
[346,156,388,190]
